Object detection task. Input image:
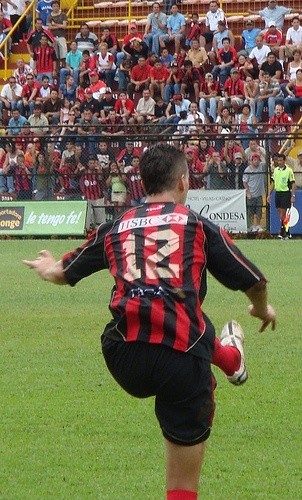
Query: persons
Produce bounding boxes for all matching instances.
[22,141,277,500]
[0,0,302,240]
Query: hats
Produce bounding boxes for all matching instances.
[229,68,240,73]
[246,19,254,25]
[252,154,260,161]
[129,22,138,30]
[169,95,182,102]
[235,152,242,158]
[204,72,214,80]
[268,21,277,28]
[85,88,94,94]
[90,72,98,76]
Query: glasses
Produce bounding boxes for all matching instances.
[8,78,135,145]
[236,158,241,160]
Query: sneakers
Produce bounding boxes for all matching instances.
[221,320,248,387]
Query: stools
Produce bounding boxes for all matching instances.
[86,0,302,28]
